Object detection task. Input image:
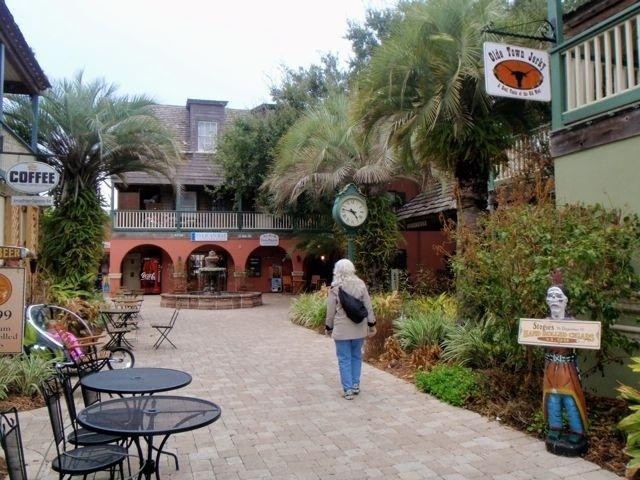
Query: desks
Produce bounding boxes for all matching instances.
[80,368,192,471]
[291,280,307,296]
[101,299,143,328]
[77,396,221,480]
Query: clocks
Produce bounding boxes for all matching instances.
[332,182,371,231]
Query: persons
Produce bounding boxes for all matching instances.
[324,258,377,400]
[273,267,281,278]
[148,270,155,288]
[103,276,109,292]
[541,284,590,444]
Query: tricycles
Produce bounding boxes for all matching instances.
[26,304,135,376]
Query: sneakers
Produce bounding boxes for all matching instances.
[342,390,353,400]
[353,383,360,393]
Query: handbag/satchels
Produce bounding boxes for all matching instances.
[337,287,367,323]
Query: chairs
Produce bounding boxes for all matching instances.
[309,275,321,292]
[0,301,182,480]
